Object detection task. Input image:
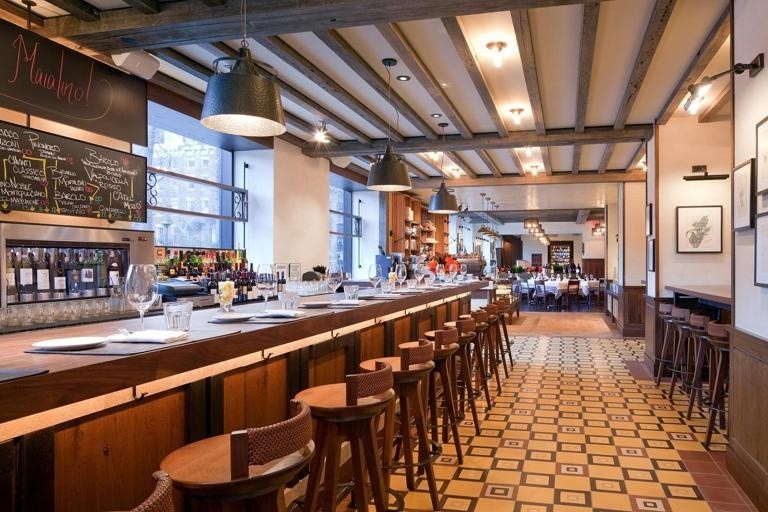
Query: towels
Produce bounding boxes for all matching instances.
[333,299,367,307]
[109,328,187,344]
[256,309,305,319]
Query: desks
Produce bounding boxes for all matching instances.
[664,284,731,322]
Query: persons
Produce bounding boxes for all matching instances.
[482,259,498,278]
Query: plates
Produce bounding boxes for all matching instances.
[359,295,374,300]
[30,336,111,351]
[302,301,332,308]
[212,314,255,322]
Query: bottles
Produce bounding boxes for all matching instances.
[218,273,232,281]
[6,248,128,295]
[276,271,281,291]
[272,274,277,300]
[257,274,264,301]
[232,274,255,306]
[157,242,186,278]
[262,273,267,301]
[281,271,287,292]
[187,241,254,273]
[190,274,217,295]
[266,273,273,301]
[542,259,581,274]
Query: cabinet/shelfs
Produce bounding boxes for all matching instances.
[418,203,448,257]
[388,193,421,257]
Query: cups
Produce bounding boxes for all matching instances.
[218,282,235,314]
[381,280,393,296]
[278,293,298,311]
[424,273,434,289]
[457,275,464,282]
[344,285,359,303]
[4,297,126,327]
[162,301,192,333]
[287,281,329,295]
[406,279,418,292]
[466,274,473,281]
[488,281,493,287]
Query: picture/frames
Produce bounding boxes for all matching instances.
[754,211,768,288]
[676,205,723,252]
[756,115,768,196]
[647,238,655,272]
[732,157,755,232]
[644,203,652,236]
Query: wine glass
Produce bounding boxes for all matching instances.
[324,262,343,303]
[532,274,594,280]
[368,264,382,294]
[459,264,467,282]
[126,264,159,336]
[395,263,408,291]
[449,264,458,284]
[414,268,426,291]
[255,264,277,315]
[435,264,445,286]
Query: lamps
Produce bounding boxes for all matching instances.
[684,51,765,115]
[428,122,458,214]
[485,41,508,68]
[366,57,412,192]
[478,193,499,240]
[313,119,331,144]
[530,165,538,175]
[524,218,550,245]
[683,163,730,181]
[510,107,522,123]
[199,0,287,138]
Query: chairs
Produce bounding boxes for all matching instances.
[424,318,479,443]
[535,280,552,311]
[130,470,176,511]
[656,303,687,385]
[294,360,396,511]
[459,304,508,395]
[491,300,516,377]
[359,339,442,511]
[666,307,703,405]
[520,282,534,304]
[159,400,317,512]
[594,278,604,306]
[395,325,463,475]
[685,313,727,430]
[443,309,491,413]
[700,322,730,445]
[567,281,580,310]
